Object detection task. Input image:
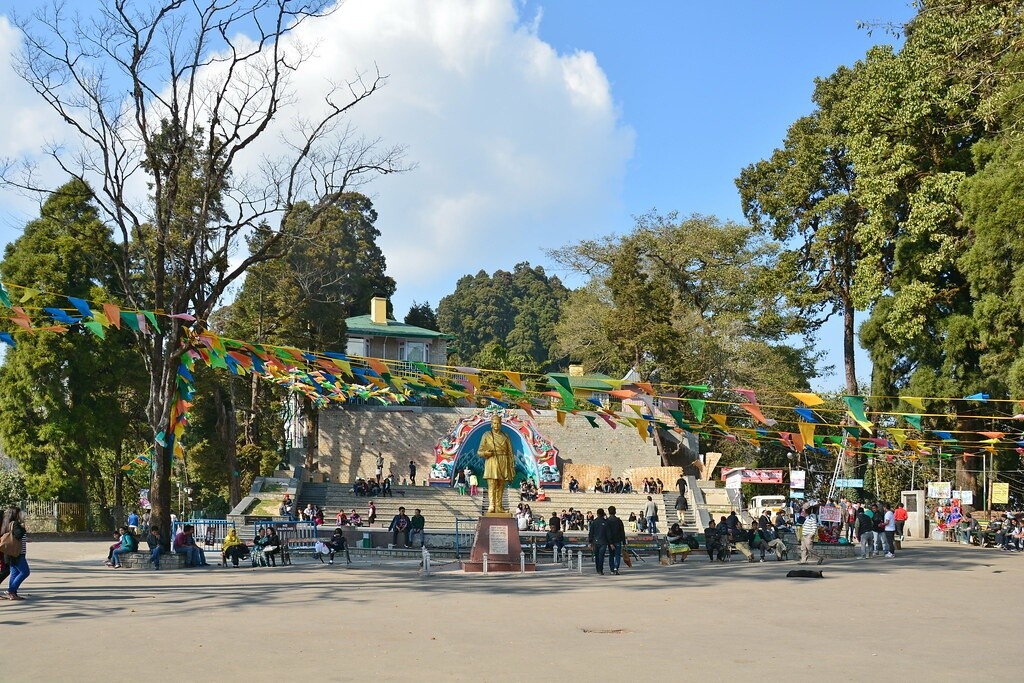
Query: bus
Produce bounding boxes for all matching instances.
[749,494,786,521]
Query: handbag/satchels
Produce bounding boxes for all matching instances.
[263,545,277,551]
[684,536,699,549]
[0,521,23,557]
[622,544,632,567]
[315,541,330,554]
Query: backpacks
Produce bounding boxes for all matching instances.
[124,534,139,552]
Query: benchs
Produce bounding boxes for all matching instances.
[220,538,284,566]
[284,537,353,565]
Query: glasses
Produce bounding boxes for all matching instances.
[334,532,339,534]
[401,510,405,512]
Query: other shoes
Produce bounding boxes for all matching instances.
[778,557,783,560]
[253,563,276,567]
[673,559,684,563]
[187,563,196,568]
[963,542,968,544]
[857,551,895,559]
[982,543,1017,551]
[197,563,210,567]
[798,562,809,565]
[783,549,792,554]
[147,559,151,563]
[597,570,603,575]
[327,561,333,564]
[391,544,427,549]
[770,550,775,555]
[759,559,766,562]
[103,560,121,569]
[898,535,904,541]
[610,568,620,574]
[0,596,7,599]
[233,565,239,568]
[749,555,754,562]
[710,558,723,563]
[155,566,159,570]
[4,591,23,600]
[817,557,823,564]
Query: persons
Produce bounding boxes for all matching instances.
[5,507,30,600]
[0,510,12,600]
[127,510,138,535]
[187,526,210,567]
[376,452,384,476]
[281,459,1024,574]
[169,509,178,540]
[106,527,134,568]
[264,527,281,567]
[477,416,515,513]
[252,527,268,566]
[146,526,164,570]
[174,525,198,566]
[222,528,247,568]
[142,510,151,536]
[104,532,123,562]
